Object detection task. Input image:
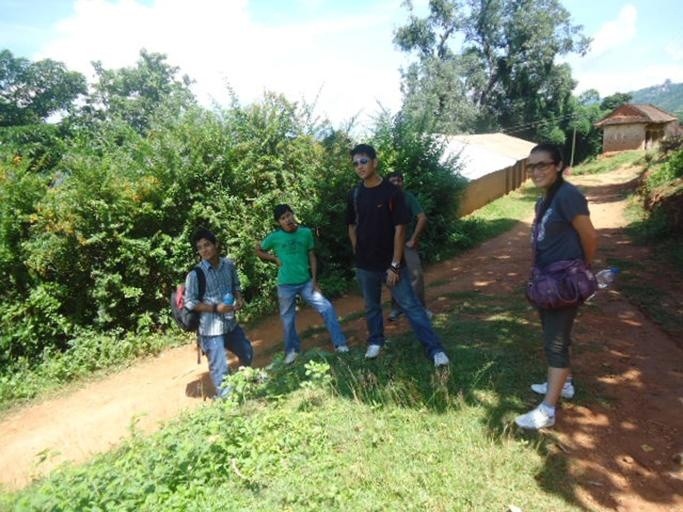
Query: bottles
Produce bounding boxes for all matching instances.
[223,291,235,320]
[585,267,621,301]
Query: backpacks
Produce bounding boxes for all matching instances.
[170,267,207,332]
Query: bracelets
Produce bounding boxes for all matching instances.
[389,261,400,274]
[213,303,218,313]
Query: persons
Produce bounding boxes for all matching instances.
[183,229,255,397]
[256,204,348,365]
[345,143,449,367]
[515,143,598,432]
[387,171,435,323]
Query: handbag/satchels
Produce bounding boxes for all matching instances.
[524,257,599,311]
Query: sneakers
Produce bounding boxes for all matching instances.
[364,344,381,358]
[514,404,554,430]
[283,351,298,365]
[425,309,433,320]
[334,346,349,353]
[388,311,401,321]
[433,352,450,368]
[531,381,573,399]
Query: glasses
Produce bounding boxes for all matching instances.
[351,158,373,167]
[525,161,557,173]
